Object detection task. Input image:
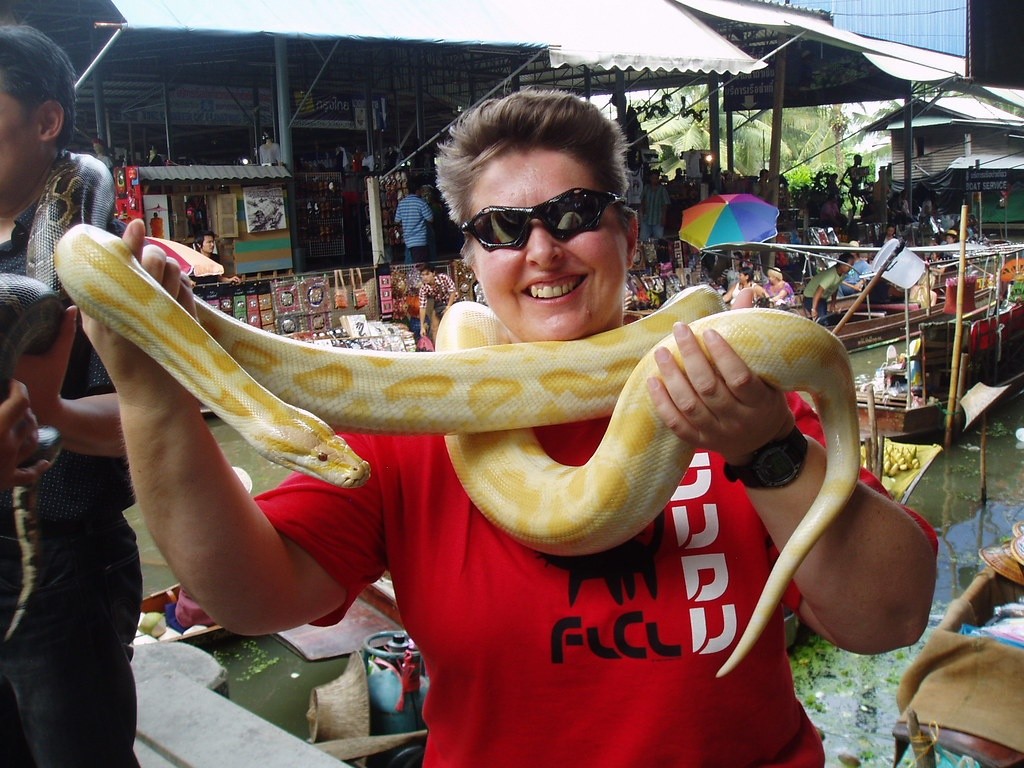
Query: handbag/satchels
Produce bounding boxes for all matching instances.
[377,254,391,275]
[334,269,348,309]
[349,268,368,309]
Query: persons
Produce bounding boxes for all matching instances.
[188,229,242,288]
[0,25,140,768]
[713,252,795,310]
[876,224,905,255]
[802,252,864,327]
[81,86,938,768]
[418,263,460,341]
[393,178,433,264]
[260,129,281,166]
[931,229,959,270]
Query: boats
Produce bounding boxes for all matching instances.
[623,241,1024,445]
[129,582,241,647]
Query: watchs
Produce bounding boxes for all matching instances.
[724,426,808,490]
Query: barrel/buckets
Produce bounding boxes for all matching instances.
[870,239,925,289]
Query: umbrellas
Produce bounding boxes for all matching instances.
[679,194,779,251]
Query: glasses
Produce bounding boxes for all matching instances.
[461,187,627,252]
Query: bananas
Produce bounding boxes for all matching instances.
[860,445,922,477]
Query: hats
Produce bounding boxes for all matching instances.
[947,230,957,236]
